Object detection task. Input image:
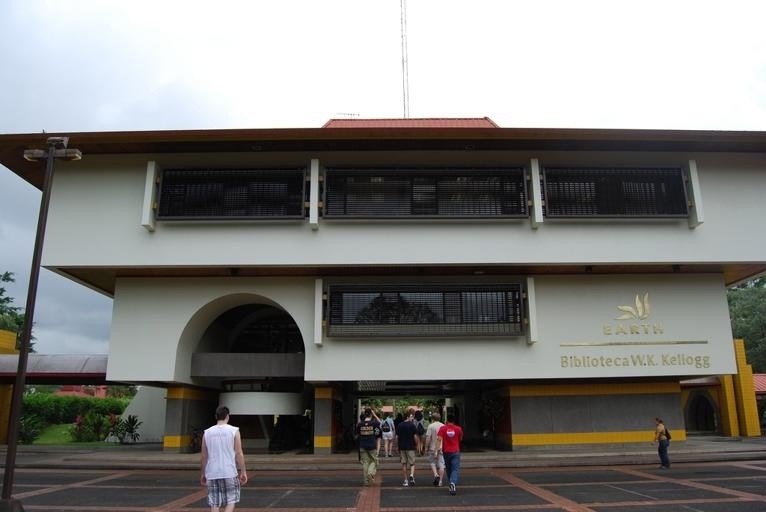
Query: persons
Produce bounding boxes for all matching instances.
[651,418,672,469]
[434,413,463,495]
[199,406,247,512]
[356,408,425,461]
[425,412,445,487]
[354,408,382,485]
[396,413,419,487]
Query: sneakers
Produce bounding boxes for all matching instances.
[433,473,440,486]
[389,453,393,457]
[368,473,375,481]
[384,453,388,457]
[448,482,456,496]
[409,473,415,487]
[360,480,369,485]
[400,480,409,487]
[438,481,442,486]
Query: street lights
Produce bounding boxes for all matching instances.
[0,137,84,511]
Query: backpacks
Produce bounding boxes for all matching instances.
[382,421,390,432]
[418,423,424,435]
